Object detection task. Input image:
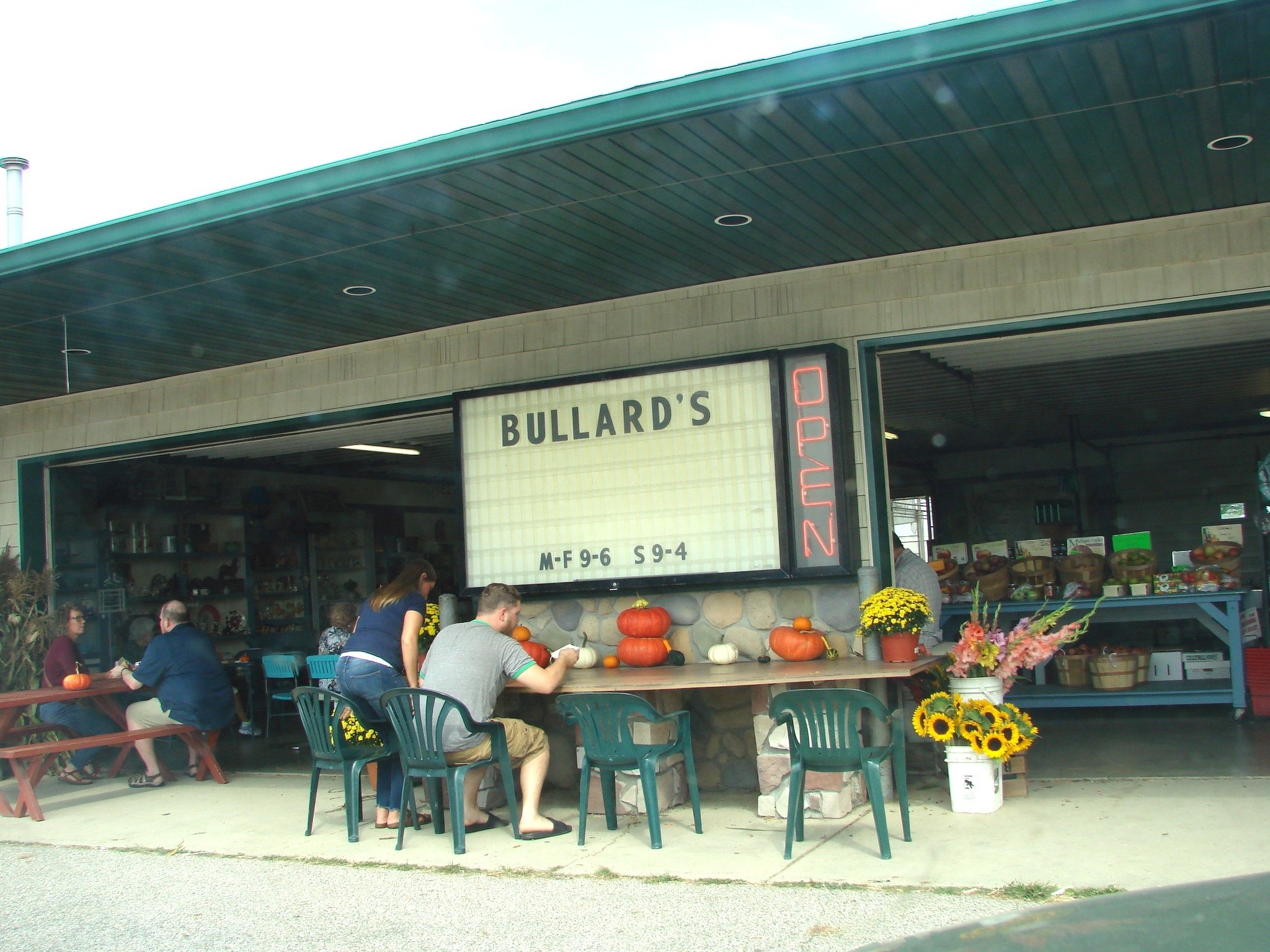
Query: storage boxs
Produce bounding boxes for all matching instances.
[1181,651,1223,662]
[1244,647,1270,716]
[1184,661,1230,680]
[1147,649,1183,682]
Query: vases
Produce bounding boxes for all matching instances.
[881,630,919,663]
[948,674,1004,704]
[418,652,427,671]
[945,745,1004,813]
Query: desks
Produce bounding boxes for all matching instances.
[0,678,176,788]
[941,585,1250,721]
[503,656,944,803]
[222,661,256,738]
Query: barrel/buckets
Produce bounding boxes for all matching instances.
[1189,542,1243,586]
[1054,647,1149,685]
[928,548,1158,602]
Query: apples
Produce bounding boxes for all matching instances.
[1071,545,1092,554]
[1064,581,1092,599]
[1101,552,1154,585]
[1010,582,1042,601]
[1055,645,1149,655]
[1191,546,1238,561]
[976,549,991,559]
[937,548,951,559]
[967,555,1005,576]
[1180,568,1236,592]
[935,560,956,576]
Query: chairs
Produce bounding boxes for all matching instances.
[379,687,522,854]
[768,688,911,859]
[291,686,420,842]
[556,692,703,849]
[306,655,341,700]
[261,655,299,738]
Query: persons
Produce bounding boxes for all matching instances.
[319,602,357,710]
[892,531,943,647]
[121,617,156,665]
[418,583,579,840]
[335,559,436,829]
[40,602,120,784]
[110,600,234,789]
[232,687,262,734]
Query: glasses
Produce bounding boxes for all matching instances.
[71,615,88,622]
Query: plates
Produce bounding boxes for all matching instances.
[198,605,220,631]
[226,610,246,634]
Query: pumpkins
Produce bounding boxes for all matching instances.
[708,634,738,664]
[62,662,92,690]
[769,625,827,661]
[603,655,620,668]
[793,615,812,631]
[511,624,531,642]
[240,653,249,661]
[617,598,672,638]
[826,649,839,660]
[668,649,685,666]
[519,641,550,669]
[758,655,770,663]
[617,636,672,667]
[571,631,599,668]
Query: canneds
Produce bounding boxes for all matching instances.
[1043,581,1056,600]
[1007,583,1017,599]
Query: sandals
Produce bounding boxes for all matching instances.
[128,770,165,787]
[59,769,92,785]
[185,764,198,776]
[81,762,104,779]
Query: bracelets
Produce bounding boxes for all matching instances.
[121,668,128,678]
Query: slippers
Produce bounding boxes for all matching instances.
[388,808,431,828]
[520,813,572,840]
[375,821,387,828]
[464,810,510,834]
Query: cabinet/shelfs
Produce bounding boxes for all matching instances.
[105,500,455,645]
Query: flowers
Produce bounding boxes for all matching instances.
[854,586,936,640]
[946,579,1107,695]
[418,603,440,653]
[329,713,384,748]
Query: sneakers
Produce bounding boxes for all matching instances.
[239,720,262,735]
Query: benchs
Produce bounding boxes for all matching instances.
[0,726,230,821]
[8,722,54,737]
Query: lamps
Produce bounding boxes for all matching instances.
[337,443,424,455]
